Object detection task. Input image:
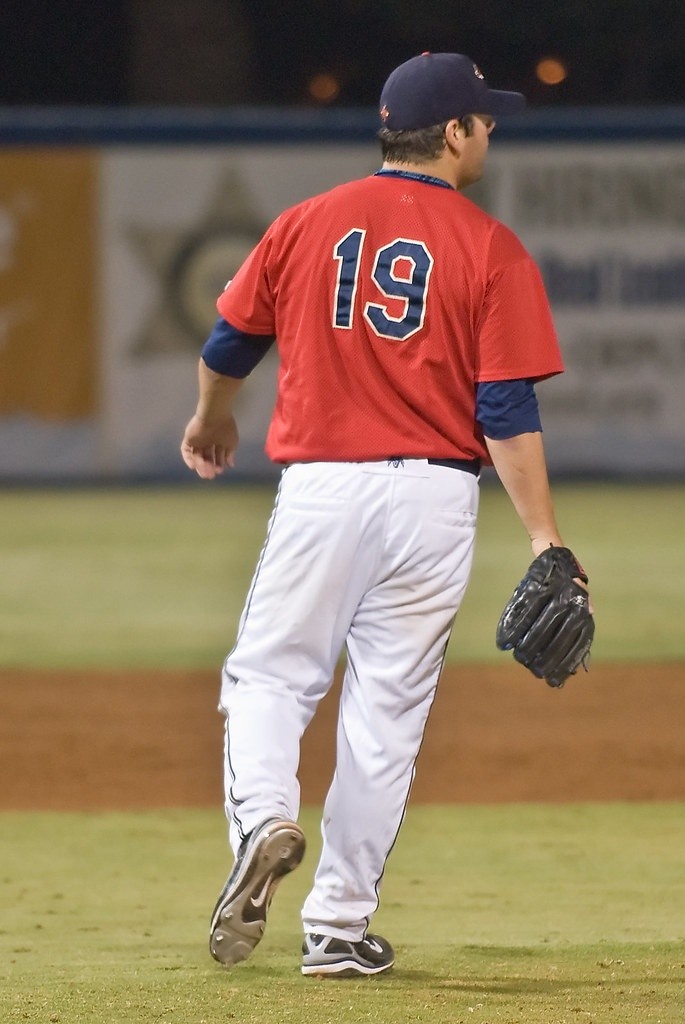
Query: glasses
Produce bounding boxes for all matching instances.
[472,112,496,136]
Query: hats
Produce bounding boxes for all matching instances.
[378,50,527,131]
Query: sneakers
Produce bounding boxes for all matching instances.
[301,933,396,976]
[208,813,307,965]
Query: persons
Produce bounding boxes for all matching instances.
[180,49,596,981]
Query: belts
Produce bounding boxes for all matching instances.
[428,456,481,477]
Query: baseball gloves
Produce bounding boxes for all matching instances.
[492,545,600,684]
